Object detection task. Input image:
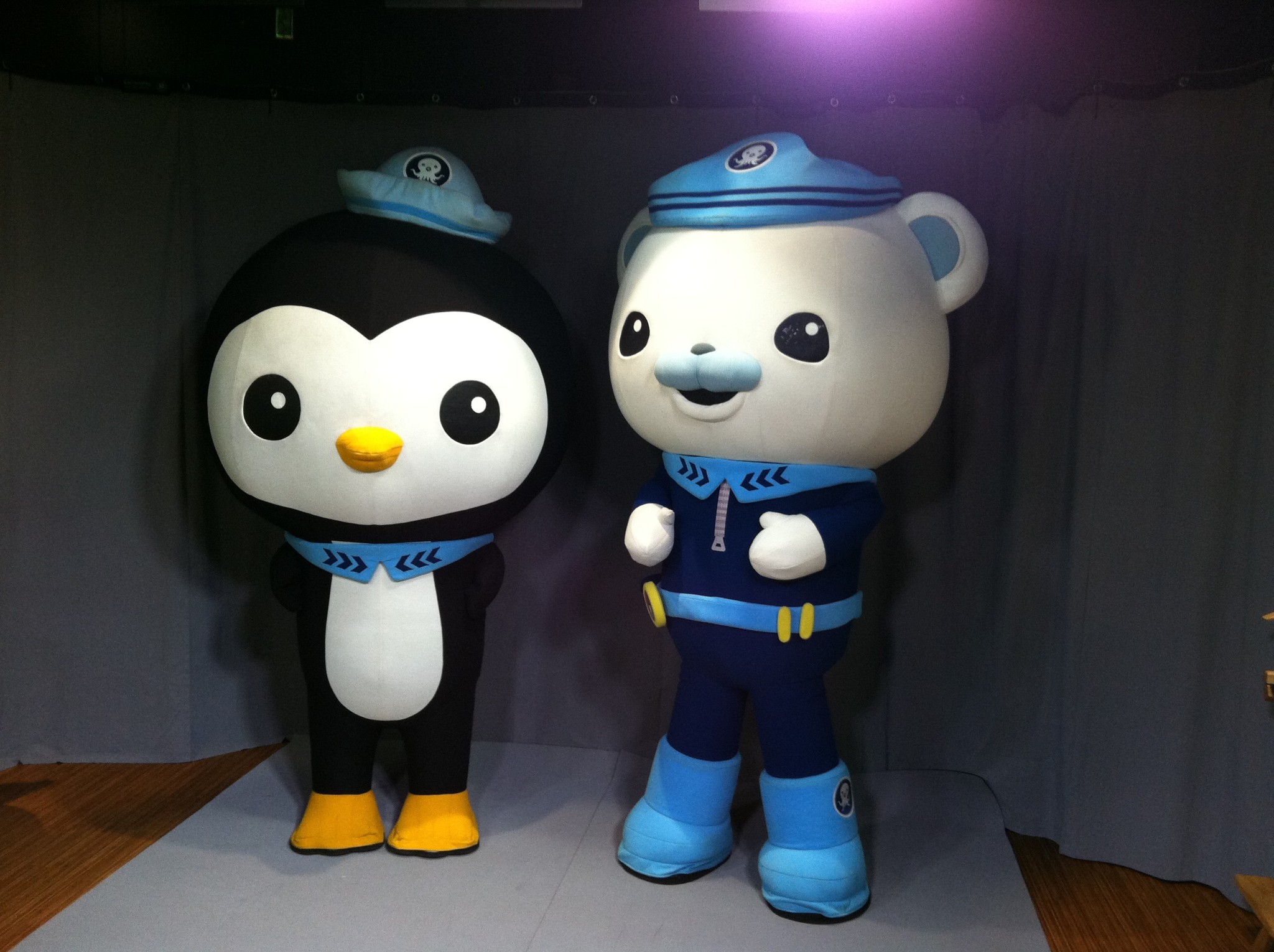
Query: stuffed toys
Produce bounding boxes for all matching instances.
[201,145,553,851]
[606,128,985,922]
